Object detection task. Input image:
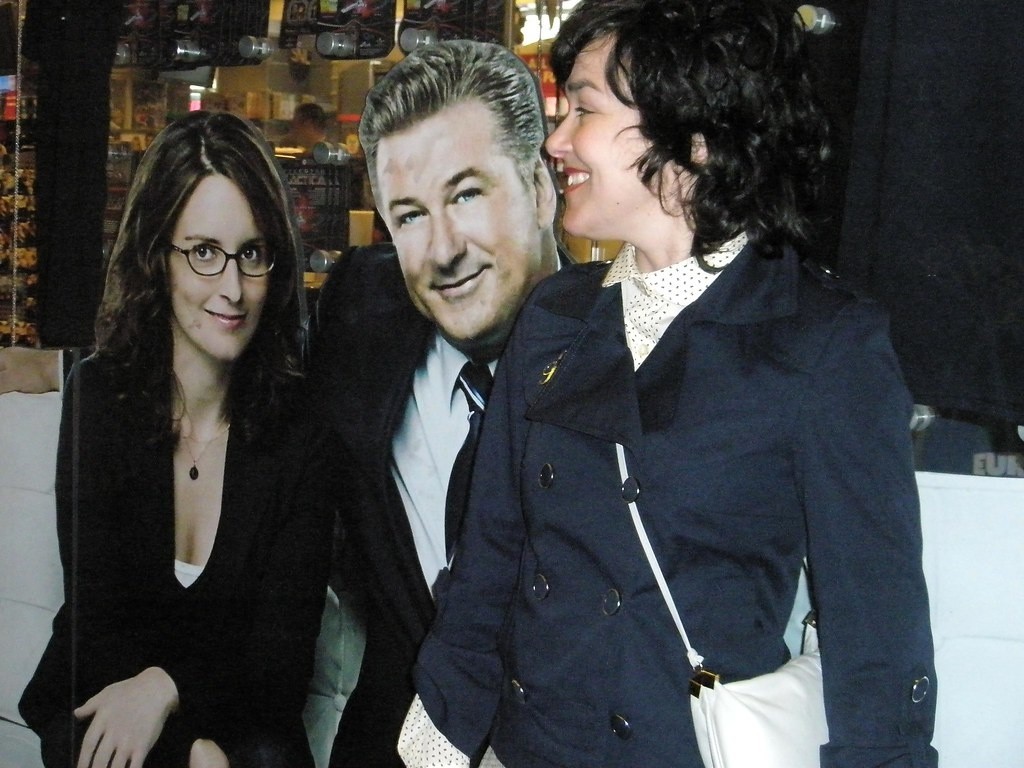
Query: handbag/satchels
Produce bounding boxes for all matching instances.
[689,609,829,768]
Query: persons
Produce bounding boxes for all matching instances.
[290,104,347,272]
[19,40,574,768]
[397,0,939,768]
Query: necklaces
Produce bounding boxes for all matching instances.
[174,414,227,481]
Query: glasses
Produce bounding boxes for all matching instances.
[166,242,279,276]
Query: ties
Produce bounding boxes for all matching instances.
[445,364,499,564]
